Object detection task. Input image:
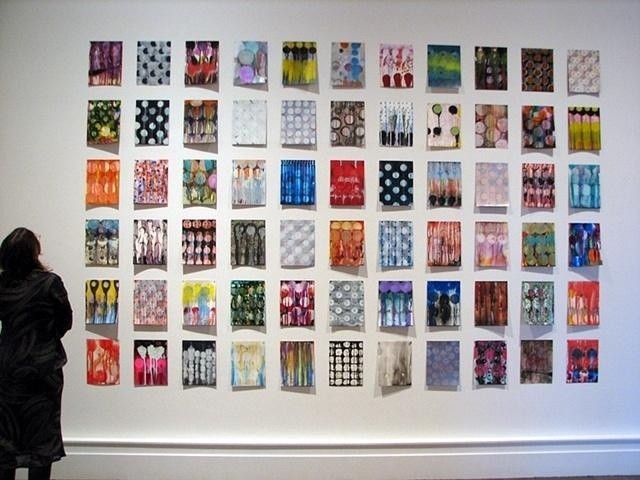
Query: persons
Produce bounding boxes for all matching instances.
[1,228,72,480]
